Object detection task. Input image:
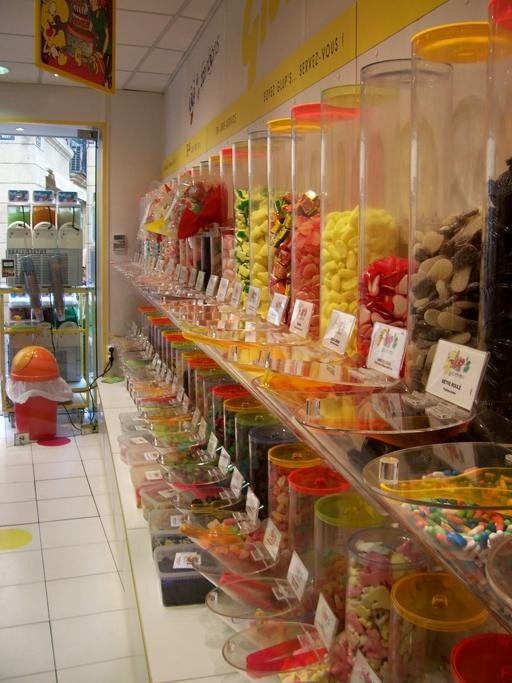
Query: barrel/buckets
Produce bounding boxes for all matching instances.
[14,380,58,442]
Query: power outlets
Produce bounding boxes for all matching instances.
[108,345,115,354]
[108,355,114,364]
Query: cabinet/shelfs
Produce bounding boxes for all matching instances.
[95,260,512,683]
[0,287,90,428]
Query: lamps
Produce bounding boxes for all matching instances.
[0,66,9,75]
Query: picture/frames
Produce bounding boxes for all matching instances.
[33,0,117,96]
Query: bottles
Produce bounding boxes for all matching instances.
[187,356,219,403]
[248,424,299,521]
[246,130,269,319]
[265,116,320,326]
[474,0,512,420]
[316,83,398,359]
[137,305,183,363]
[135,154,221,289]
[401,21,489,392]
[212,382,251,446]
[287,465,351,566]
[231,140,248,309]
[170,339,195,374]
[220,147,234,303]
[267,441,324,556]
[234,408,281,481]
[181,351,208,396]
[222,396,265,463]
[356,55,454,379]
[202,374,235,425]
[311,490,392,635]
[175,343,198,383]
[288,103,358,341]
[194,367,230,413]
[343,526,431,681]
[384,569,490,681]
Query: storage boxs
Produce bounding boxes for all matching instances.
[154,546,215,606]
[128,447,161,465]
[150,509,194,558]
[118,433,155,464]
[131,464,167,508]
[127,446,157,466]
[119,413,147,431]
[139,485,178,521]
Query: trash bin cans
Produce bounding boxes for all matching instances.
[9,345,61,438]
